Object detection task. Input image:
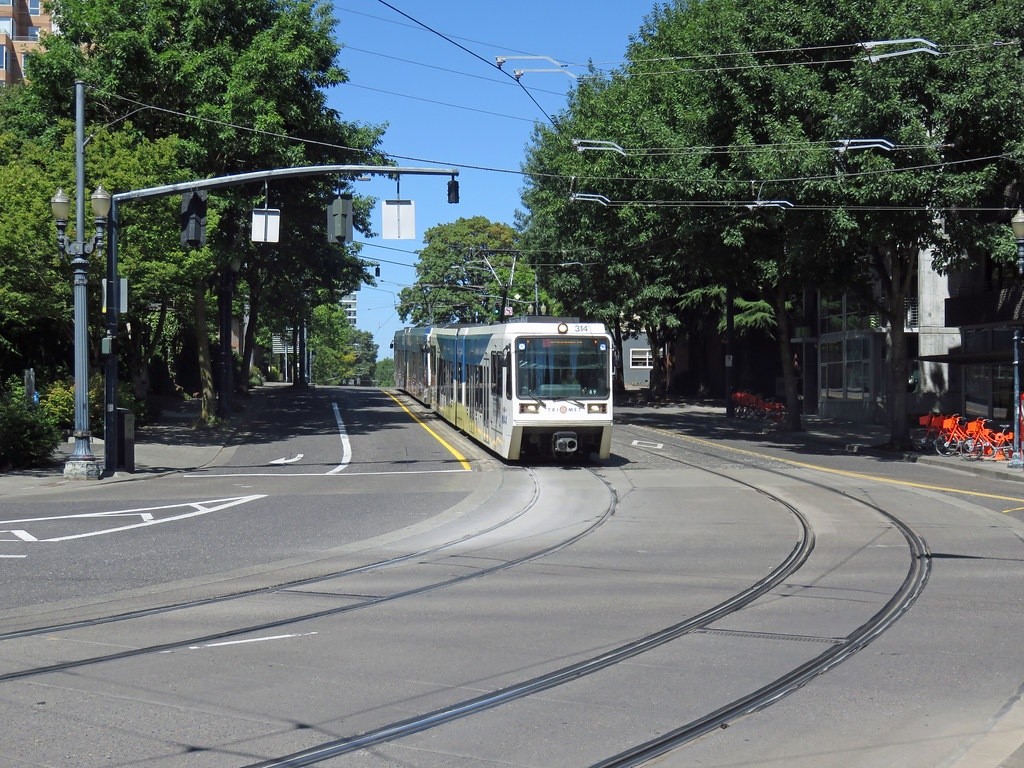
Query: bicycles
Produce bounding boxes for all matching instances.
[911,410,1014,461]
[731,390,787,427]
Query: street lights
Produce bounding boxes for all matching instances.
[51,183,109,480]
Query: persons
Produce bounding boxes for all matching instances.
[560,369,581,385]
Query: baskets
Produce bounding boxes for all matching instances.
[734,393,779,411]
[916,415,980,432]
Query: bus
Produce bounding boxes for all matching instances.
[390,316,613,464]
